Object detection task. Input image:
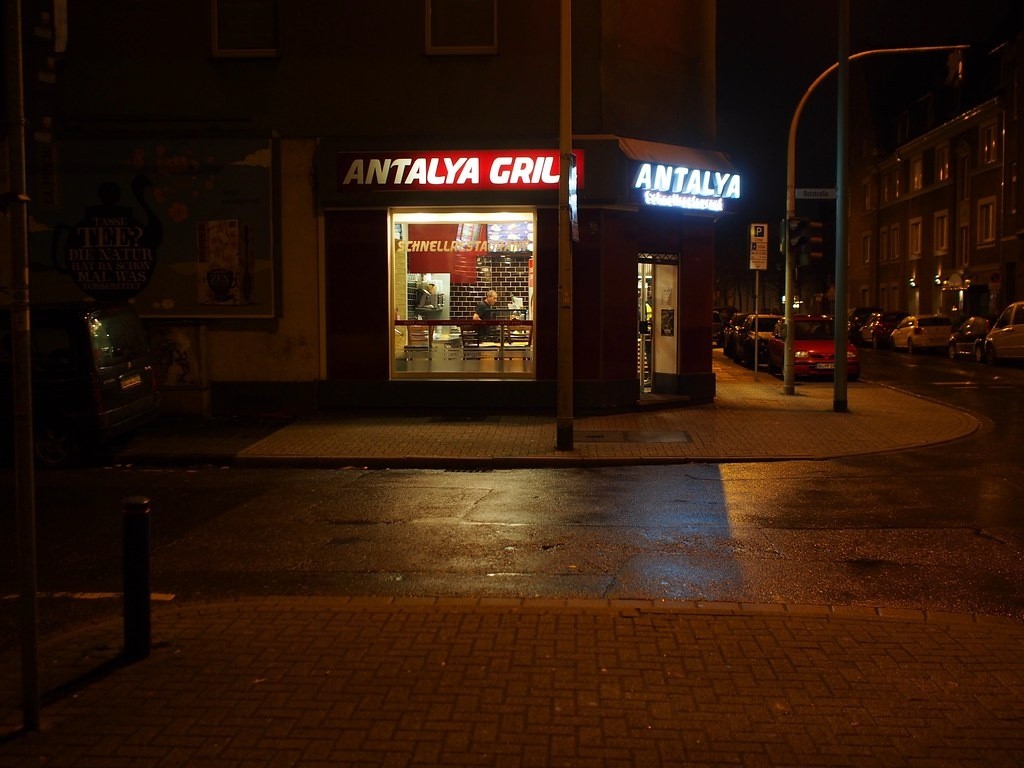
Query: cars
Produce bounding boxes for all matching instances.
[767,312,862,382]
[712,310,727,347]
[946,311,1006,361]
[846,306,911,350]
[723,312,750,356]
[888,314,956,355]
[984,301,1024,366]
[738,313,782,363]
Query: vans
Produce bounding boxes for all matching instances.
[0,298,162,469]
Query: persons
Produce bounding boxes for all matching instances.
[473,290,498,335]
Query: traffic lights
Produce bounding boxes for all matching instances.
[788,216,809,253]
[946,56,968,85]
[907,276,916,289]
[933,273,941,287]
[798,216,824,267]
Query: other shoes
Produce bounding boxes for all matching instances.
[644,379,652,387]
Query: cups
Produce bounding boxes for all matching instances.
[206,269,233,302]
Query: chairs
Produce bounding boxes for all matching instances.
[404,325,433,360]
[462,325,498,360]
[500,325,532,360]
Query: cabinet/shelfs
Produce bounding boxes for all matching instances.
[407,281,427,320]
